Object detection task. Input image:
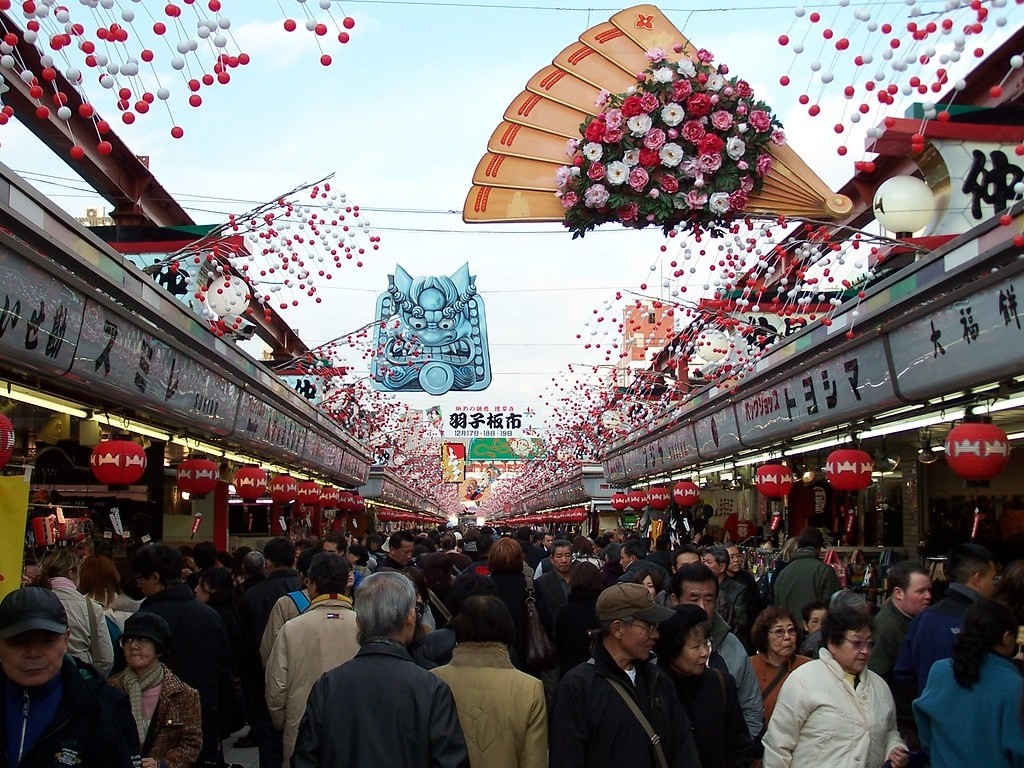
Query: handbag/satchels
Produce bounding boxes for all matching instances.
[525,602,557,672]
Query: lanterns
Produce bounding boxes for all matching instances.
[269,476,364,511]
[0,410,15,471]
[90,434,147,491]
[945,415,1008,489]
[177,454,219,501]
[377,506,447,528]
[485,509,588,525]
[611,481,700,512]
[756,460,793,502]
[825,440,874,498]
[233,463,267,504]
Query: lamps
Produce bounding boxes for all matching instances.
[207,275,250,320]
[918,421,1024,454]
[557,448,571,464]
[369,430,387,448]
[323,386,349,414]
[880,435,901,472]
[601,409,622,430]
[729,468,741,486]
[872,174,937,239]
[258,461,288,474]
[783,431,855,458]
[671,470,699,481]
[78,419,100,447]
[169,432,225,457]
[228,497,296,504]
[694,329,730,365]
[916,429,938,464]
[968,386,1024,416]
[223,450,262,467]
[89,408,175,441]
[394,453,406,466]
[0,398,18,415]
[0,377,89,419]
[801,453,816,483]
[289,469,346,490]
[853,402,968,440]
[698,461,734,475]
[734,448,784,467]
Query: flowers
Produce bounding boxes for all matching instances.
[552,38,787,241]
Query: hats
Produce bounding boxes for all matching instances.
[595,582,677,623]
[120,612,172,649]
[0,587,68,637]
[797,527,823,549]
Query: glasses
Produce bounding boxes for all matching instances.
[770,627,798,637]
[135,573,148,584]
[618,622,661,633]
[119,637,149,649]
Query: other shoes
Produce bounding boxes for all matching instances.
[233,737,259,748]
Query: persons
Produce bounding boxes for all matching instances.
[0,525,1024,768]
